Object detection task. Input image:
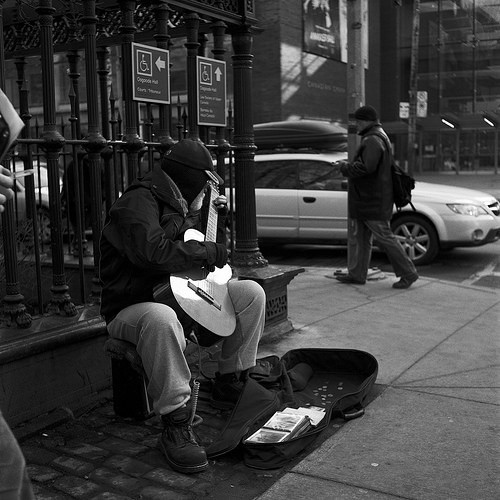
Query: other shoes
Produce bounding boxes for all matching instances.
[336,273,366,284]
[393,273,418,288]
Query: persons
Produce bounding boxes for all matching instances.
[0,164,35,500]
[63,126,119,228]
[334,106,419,290]
[99,139,266,473]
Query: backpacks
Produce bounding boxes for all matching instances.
[391,163,416,212]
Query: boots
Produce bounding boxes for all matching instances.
[211,371,245,410]
[156,404,209,473]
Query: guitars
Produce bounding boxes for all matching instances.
[151,171,238,348]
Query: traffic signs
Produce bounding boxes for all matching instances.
[195,54,229,129]
[131,42,171,105]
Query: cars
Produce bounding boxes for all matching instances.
[11,160,125,247]
[212,120,499,265]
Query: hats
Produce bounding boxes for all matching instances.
[162,138,224,184]
[349,105,378,121]
